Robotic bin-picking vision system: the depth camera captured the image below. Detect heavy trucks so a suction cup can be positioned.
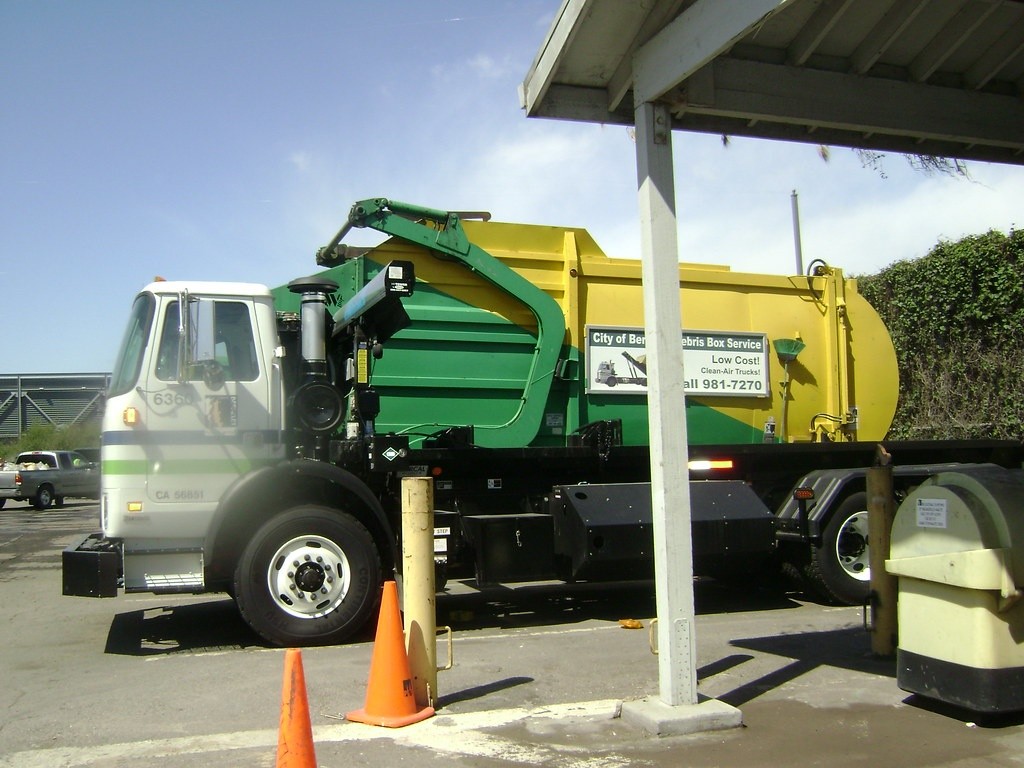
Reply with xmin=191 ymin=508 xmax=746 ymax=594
xmin=61 ymin=197 xmax=997 ymax=647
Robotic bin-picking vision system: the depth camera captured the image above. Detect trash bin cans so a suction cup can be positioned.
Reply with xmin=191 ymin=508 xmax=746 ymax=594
xmin=884 ymin=470 xmax=1024 ymax=713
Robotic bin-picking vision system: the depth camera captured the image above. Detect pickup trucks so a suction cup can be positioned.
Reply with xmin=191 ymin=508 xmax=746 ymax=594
xmin=0 ymin=450 xmax=100 ymax=512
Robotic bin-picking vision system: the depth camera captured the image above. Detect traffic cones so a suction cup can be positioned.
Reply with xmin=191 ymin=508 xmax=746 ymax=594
xmin=270 ymin=646 xmax=320 ymax=767
xmin=344 ymin=579 xmax=437 ymax=731
